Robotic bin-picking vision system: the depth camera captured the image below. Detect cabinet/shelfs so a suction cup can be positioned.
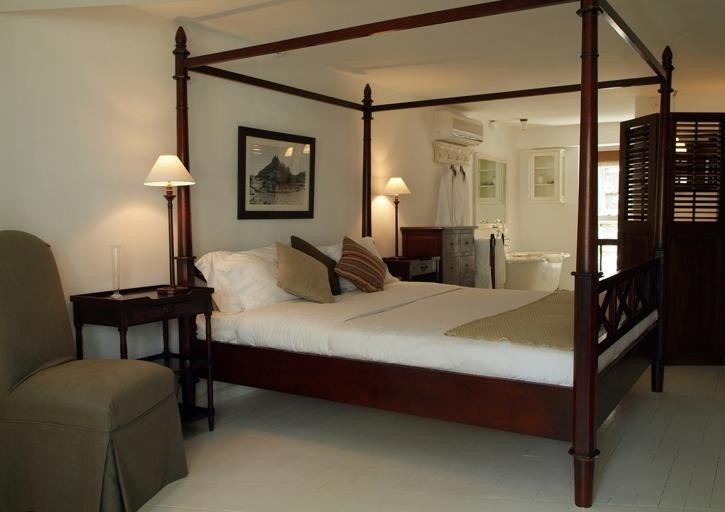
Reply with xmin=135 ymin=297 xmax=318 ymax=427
xmin=400 ymin=225 xmax=478 ymax=287
xmin=528 ymin=148 xmax=566 ymax=203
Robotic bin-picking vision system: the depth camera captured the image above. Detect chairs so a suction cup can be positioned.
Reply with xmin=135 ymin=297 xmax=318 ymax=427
xmin=0 ymin=231 xmax=188 ymax=512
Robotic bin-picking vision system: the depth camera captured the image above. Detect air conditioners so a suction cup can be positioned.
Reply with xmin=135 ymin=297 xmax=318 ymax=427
xmin=433 ymin=110 xmax=483 ymax=146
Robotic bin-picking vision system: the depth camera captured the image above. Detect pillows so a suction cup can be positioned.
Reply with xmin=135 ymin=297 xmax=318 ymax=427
xmin=291 ymin=234 xmax=341 ymax=295
xmin=276 ymin=241 xmax=334 ymax=304
xmin=194 ymin=242 xmax=299 ymax=313
xmin=319 ymin=236 xmax=399 ymax=291
xmin=334 ymin=236 xmax=387 ymax=292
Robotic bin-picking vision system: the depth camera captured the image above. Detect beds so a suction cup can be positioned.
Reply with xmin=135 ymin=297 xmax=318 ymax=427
xmin=172 ymin=0 xmax=674 ymax=507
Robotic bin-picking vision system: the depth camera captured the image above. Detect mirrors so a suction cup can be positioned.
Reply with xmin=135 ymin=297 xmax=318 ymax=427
xmin=473 ymin=152 xmax=509 ymax=226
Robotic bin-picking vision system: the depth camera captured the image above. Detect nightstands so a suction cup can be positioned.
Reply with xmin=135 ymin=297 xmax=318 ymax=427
xmin=382 ymin=256 xmax=440 ymax=283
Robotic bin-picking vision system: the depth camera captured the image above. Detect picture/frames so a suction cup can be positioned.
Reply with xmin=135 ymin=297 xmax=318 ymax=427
xmin=238 ymin=125 xmax=315 ymax=219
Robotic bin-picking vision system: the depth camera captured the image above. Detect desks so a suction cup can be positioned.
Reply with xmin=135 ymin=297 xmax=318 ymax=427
xmin=69 ymin=284 xmax=215 ymax=431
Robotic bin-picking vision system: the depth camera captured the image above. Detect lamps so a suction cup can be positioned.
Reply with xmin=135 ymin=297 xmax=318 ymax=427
xmin=384 ymin=177 xmax=411 ymax=259
xmin=145 ymin=155 xmax=195 ymax=293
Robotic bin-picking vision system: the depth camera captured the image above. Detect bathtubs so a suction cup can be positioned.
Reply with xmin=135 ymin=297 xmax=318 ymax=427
xmin=504 ymin=251 xmax=571 ymax=290
